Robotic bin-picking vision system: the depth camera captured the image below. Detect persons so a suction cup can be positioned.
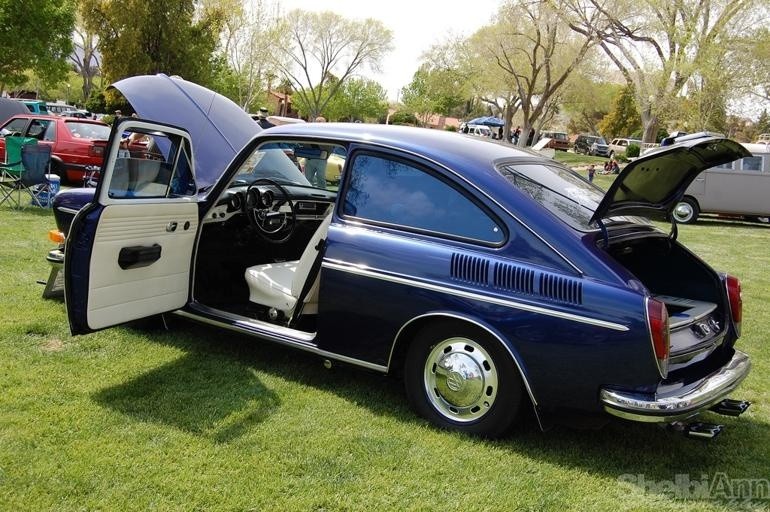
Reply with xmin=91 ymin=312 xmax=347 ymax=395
xmin=120 ymin=130 xmax=166 ymax=161
xmin=497 ymin=126 xmax=536 ymax=146
xmin=587 ymin=161 xmax=620 ymax=183
xmin=112 ymin=110 xmax=122 ymax=126
xmin=304 ymin=116 xmax=331 ymax=189
xmin=255 ymin=107 xmax=276 ymax=129
xmin=464 ymin=125 xmax=469 ymax=134
xmin=459 ymin=128 xmax=463 ymax=133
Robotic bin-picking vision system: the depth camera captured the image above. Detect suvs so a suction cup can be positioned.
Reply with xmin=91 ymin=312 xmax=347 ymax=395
xmin=607 ymin=138 xmax=642 ymax=159
xmin=634 ymin=138 xmax=769 ymax=225
xmin=573 ymin=134 xmax=609 ymax=157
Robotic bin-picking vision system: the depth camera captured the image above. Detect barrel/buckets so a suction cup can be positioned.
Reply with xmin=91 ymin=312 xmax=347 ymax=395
xmin=33 ymin=190 xmax=49 ymax=206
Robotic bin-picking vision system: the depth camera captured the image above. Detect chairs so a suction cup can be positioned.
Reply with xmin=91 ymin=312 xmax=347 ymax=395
xmin=244 ymin=210 xmax=334 ymax=324
xmin=0 ymin=136 xmax=50 ymax=213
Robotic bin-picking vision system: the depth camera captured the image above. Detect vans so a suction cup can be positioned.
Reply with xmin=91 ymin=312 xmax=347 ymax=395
xmin=541 ymin=131 xmax=571 ymax=151
xmin=462 ymin=125 xmax=493 ymax=139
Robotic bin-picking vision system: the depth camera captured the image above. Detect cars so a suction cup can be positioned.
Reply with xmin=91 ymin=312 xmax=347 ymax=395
xmin=49 ymin=73 xmax=756 ymax=440
xmin=20 ymin=99 xmax=88 ymax=119
xmin=2 ymin=113 xmax=130 ymax=187
xmin=2 ymin=98 xmax=46 ymax=166
xmin=126 ymin=130 xmax=165 ymax=162
